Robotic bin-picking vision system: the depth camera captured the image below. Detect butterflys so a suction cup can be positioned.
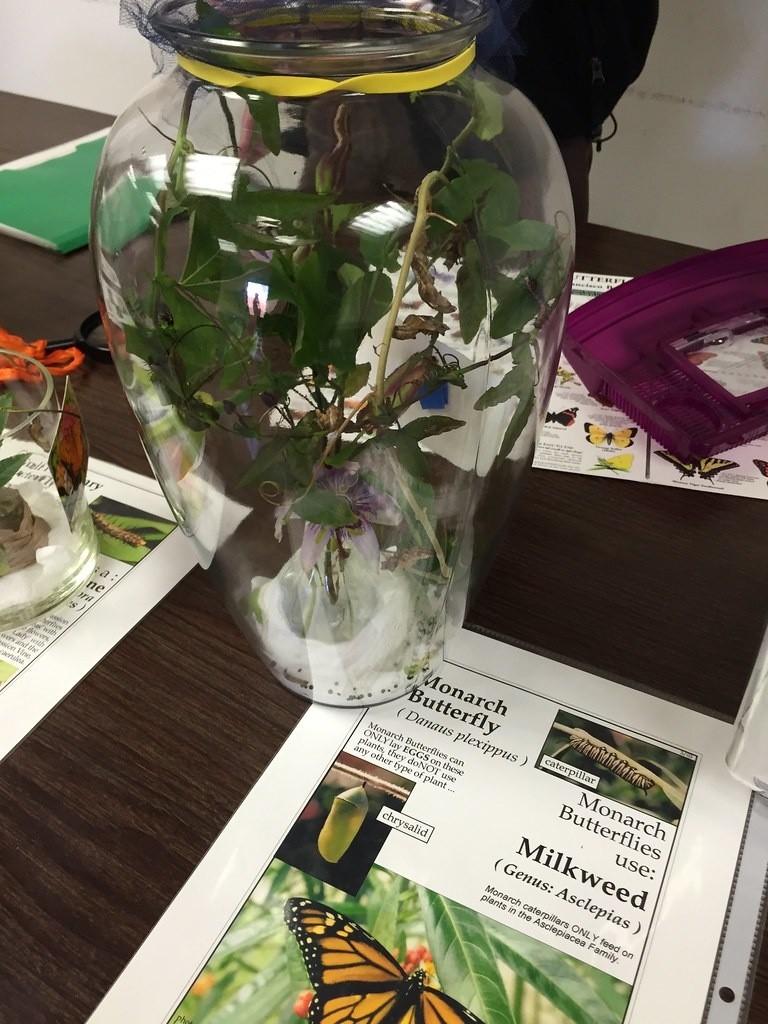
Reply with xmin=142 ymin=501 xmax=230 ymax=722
xmin=283 ymin=896 xmax=486 ymax=1024
xmin=541 ymin=335 xmax=767 ymax=485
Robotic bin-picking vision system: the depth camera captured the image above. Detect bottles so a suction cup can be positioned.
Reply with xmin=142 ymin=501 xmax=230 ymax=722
xmin=89 ymin=5 xmax=576 ymax=708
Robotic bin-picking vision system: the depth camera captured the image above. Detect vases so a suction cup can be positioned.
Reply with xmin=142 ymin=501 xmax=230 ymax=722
xmin=83 ymin=0 xmax=582 ymax=714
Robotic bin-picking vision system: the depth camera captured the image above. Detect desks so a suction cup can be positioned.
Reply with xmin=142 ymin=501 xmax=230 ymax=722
xmin=0 ymin=86 xmax=768 ymax=1024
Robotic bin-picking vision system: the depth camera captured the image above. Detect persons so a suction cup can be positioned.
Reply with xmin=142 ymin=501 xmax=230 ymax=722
xmin=299 ymin=0 xmax=660 ymax=223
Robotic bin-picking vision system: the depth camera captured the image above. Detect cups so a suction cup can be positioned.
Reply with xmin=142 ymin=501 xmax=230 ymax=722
xmin=0 ymin=350 xmax=99 ymax=632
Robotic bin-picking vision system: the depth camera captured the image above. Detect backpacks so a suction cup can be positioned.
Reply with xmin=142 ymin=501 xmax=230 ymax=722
xmin=427 ymin=0 xmax=660 ymax=153
